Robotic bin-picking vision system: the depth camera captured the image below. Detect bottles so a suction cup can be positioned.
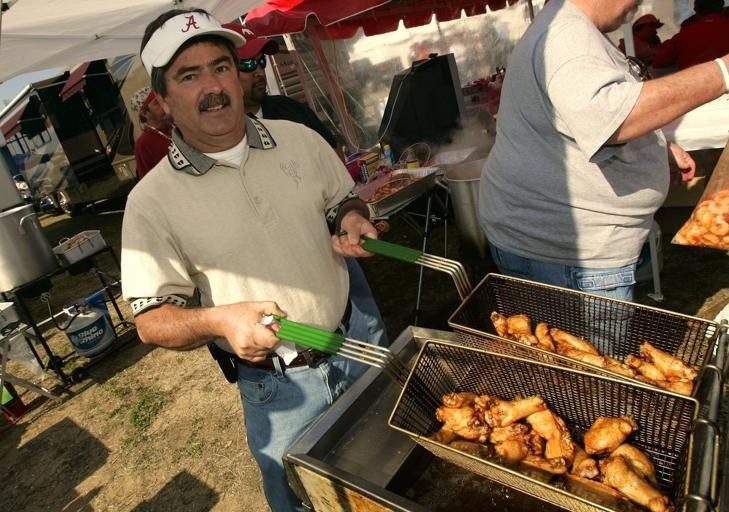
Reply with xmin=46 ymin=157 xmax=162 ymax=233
xmin=407 ymin=159 xmax=419 ymax=169
xmin=384 ymin=145 xmax=396 ymax=167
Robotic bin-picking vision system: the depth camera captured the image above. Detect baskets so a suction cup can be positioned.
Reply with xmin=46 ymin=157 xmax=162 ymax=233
xmin=388 ymin=340 xmax=700 ymax=512
xmin=447 ymin=271 xmax=720 ymax=399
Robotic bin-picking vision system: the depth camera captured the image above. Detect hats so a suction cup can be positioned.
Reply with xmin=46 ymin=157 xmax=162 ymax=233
xmin=222 ymin=23 xmax=279 ymax=60
xmin=140 ymin=12 xmax=247 ymax=79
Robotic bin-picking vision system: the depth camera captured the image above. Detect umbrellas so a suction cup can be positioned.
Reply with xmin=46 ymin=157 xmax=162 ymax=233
xmin=245 ymin=0 xmax=519 ymax=152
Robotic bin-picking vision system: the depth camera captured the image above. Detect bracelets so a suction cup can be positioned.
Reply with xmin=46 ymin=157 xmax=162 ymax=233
xmin=715 ymin=57 xmax=729 ymax=93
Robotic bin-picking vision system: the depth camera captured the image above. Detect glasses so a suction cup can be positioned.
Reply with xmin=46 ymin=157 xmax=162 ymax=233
xmin=237 ymin=56 xmax=266 ymax=72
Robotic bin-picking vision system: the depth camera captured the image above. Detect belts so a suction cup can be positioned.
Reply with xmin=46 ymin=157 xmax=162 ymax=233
xmin=236 ymin=294 xmax=352 ymax=369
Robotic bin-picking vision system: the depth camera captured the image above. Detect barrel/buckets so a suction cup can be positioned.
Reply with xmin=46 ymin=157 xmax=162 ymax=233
xmin=66 ymin=310 xmax=114 ymax=359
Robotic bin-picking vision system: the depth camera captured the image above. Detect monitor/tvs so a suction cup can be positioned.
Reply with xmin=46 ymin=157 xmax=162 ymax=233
xmin=377 ymin=53 xmax=476 ymax=160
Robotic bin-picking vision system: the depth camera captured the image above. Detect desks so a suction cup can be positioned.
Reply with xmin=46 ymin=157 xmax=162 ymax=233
xmin=4 ymin=244 xmax=170 ymax=391
xmin=354 ymin=172 xmax=477 ymax=325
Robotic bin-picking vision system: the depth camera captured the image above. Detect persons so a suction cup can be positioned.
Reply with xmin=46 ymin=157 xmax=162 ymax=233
xmin=479 ymin=0 xmax=729 ymax=356
xmin=130 ymin=86 xmax=177 ymax=180
xmin=618 ymin=14 xmax=664 ymax=63
xmin=120 ymin=8 xmax=377 ymax=512
xmin=221 ymin=23 xmax=345 ymax=166
xmin=652 ymin=0 xmax=729 ymax=70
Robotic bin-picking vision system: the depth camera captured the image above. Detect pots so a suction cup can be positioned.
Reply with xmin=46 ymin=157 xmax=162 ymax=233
xmin=0 ymin=151 xmax=60 ymax=293
xmin=434 ymin=158 xmax=498 ymax=267
